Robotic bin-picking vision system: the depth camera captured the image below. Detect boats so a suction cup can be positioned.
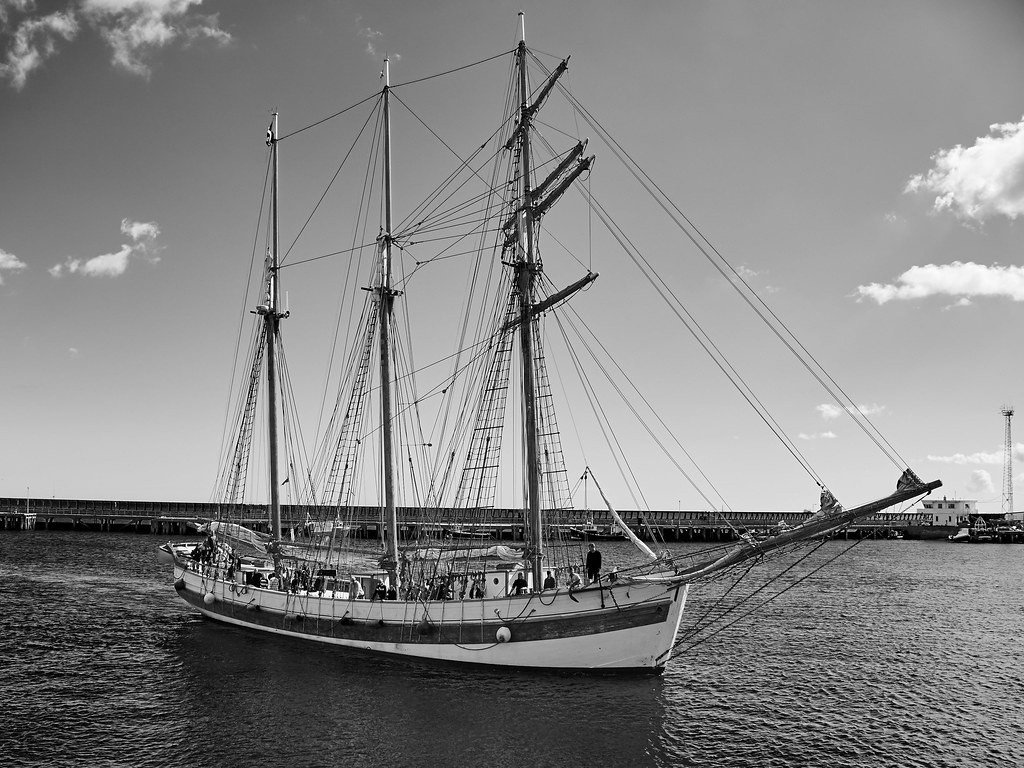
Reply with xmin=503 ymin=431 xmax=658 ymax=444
xmin=569 ymin=509 xmax=626 ymax=542
xmin=447 ymin=528 xmax=491 ymax=540
xmin=305 ymin=507 xmax=350 ymax=538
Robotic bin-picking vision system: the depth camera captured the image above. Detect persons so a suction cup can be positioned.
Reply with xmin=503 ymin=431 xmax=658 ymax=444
xmin=251 ymin=563 xmax=326 ymax=594
xmin=566 ymin=567 xmax=582 ymax=587
xmin=507 ymin=572 xmax=528 ymax=597
xmin=349 ymin=577 xmax=365 ymax=600
xmin=544 ymin=571 xmax=555 ymax=589
xmin=586 ymin=544 xmax=602 ymax=584
xmin=189 ymin=529 xmax=241 ymax=581
xmin=370 ymin=578 xmax=482 ymax=602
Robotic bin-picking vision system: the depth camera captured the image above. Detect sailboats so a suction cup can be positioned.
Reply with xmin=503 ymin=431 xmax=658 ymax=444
xmin=154 ymin=8 xmax=946 ymax=679
